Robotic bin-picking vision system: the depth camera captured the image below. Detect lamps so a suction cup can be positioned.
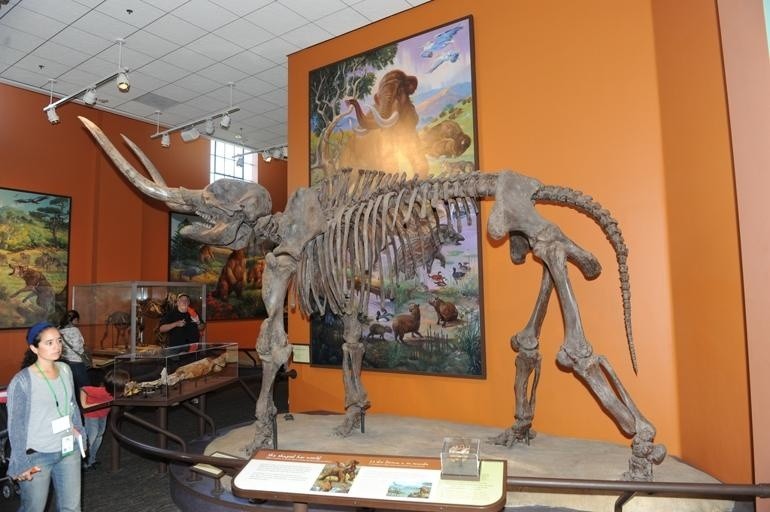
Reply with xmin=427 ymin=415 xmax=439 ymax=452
xmin=38 ymin=32 xmax=134 ymax=126
xmin=147 ymin=76 xmax=245 ymax=151
xmin=230 ymin=129 xmax=288 ymax=170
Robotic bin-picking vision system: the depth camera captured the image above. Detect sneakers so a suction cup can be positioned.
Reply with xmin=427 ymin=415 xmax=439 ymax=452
xmin=82 ymin=460 xmax=100 ymax=472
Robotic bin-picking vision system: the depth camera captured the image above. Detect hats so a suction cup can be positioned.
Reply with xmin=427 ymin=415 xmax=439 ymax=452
xmin=176 ymin=292 xmax=189 ymax=300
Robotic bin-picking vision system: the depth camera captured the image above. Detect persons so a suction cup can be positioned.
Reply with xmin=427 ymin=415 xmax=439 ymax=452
xmin=78 ymin=369 xmax=131 ymax=472
xmin=56 ymin=309 xmax=89 ymax=392
xmin=158 ymin=294 xmax=206 ymax=406
xmin=8 ymin=321 xmax=89 ymax=512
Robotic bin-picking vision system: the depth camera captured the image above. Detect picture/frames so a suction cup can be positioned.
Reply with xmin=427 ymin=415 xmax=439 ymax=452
xmin=308 ymin=14 xmax=487 ymax=380
xmin=166 ymin=209 xmax=290 ymax=324
xmin=0 ymin=186 xmax=73 ymax=330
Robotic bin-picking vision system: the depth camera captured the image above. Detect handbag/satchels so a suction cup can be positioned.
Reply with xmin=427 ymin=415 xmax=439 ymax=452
xmin=80 ymin=351 xmax=93 ymax=369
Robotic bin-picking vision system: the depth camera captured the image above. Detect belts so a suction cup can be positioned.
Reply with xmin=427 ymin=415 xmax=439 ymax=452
xmin=26 ymin=448 xmax=37 ymax=456
